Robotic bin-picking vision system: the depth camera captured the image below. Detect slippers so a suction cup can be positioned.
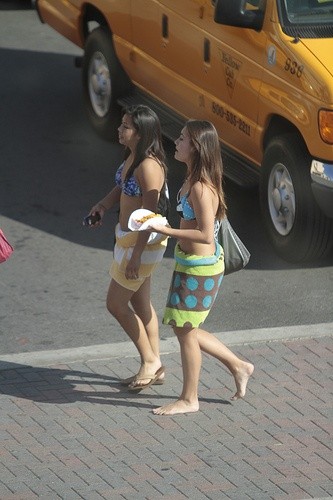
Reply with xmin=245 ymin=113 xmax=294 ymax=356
xmin=126 ymin=364 xmax=166 ymax=391
xmin=119 ymin=372 xmax=165 ymax=386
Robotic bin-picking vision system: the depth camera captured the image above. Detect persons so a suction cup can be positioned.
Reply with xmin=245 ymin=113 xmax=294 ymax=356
xmin=87 ymin=105 xmax=169 ymax=392
xmin=146 ymin=119 xmax=254 ymax=416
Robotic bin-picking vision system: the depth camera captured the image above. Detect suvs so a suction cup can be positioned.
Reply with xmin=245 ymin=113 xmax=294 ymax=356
xmin=37 ymin=0 xmax=333 ymax=264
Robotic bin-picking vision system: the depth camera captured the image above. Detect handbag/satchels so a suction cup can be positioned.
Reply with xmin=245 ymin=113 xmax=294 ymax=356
xmin=217 ymin=213 xmax=252 ymax=275
xmin=0 ymin=227 xmax=13 ymax=264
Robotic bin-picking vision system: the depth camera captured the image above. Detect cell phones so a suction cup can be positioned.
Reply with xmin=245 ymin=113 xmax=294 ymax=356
xmin=84 ymin=212 xmax=101 ymax=225
